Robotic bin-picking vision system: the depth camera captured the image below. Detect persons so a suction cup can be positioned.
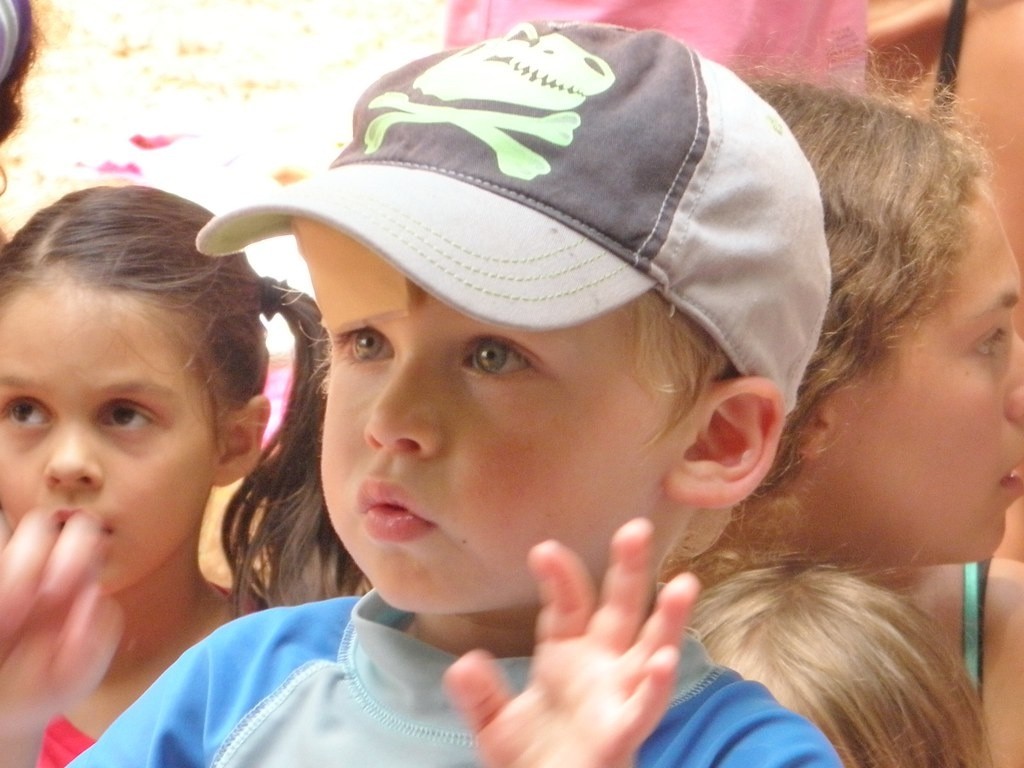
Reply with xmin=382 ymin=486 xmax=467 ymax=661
xmin=0 ymin=186 xmax=371 ymax=768
xmin=680 ymin=558 xmax=987 ymax=768
xmin=660 ymin=71 xmax=1024 ymax=768
xmin=63 ymin=21 xmax=845 ymax=768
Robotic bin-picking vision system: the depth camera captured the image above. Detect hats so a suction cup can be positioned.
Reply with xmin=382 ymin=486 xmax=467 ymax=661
xmin=191 ymin=19 xmax=833 ymax=420
